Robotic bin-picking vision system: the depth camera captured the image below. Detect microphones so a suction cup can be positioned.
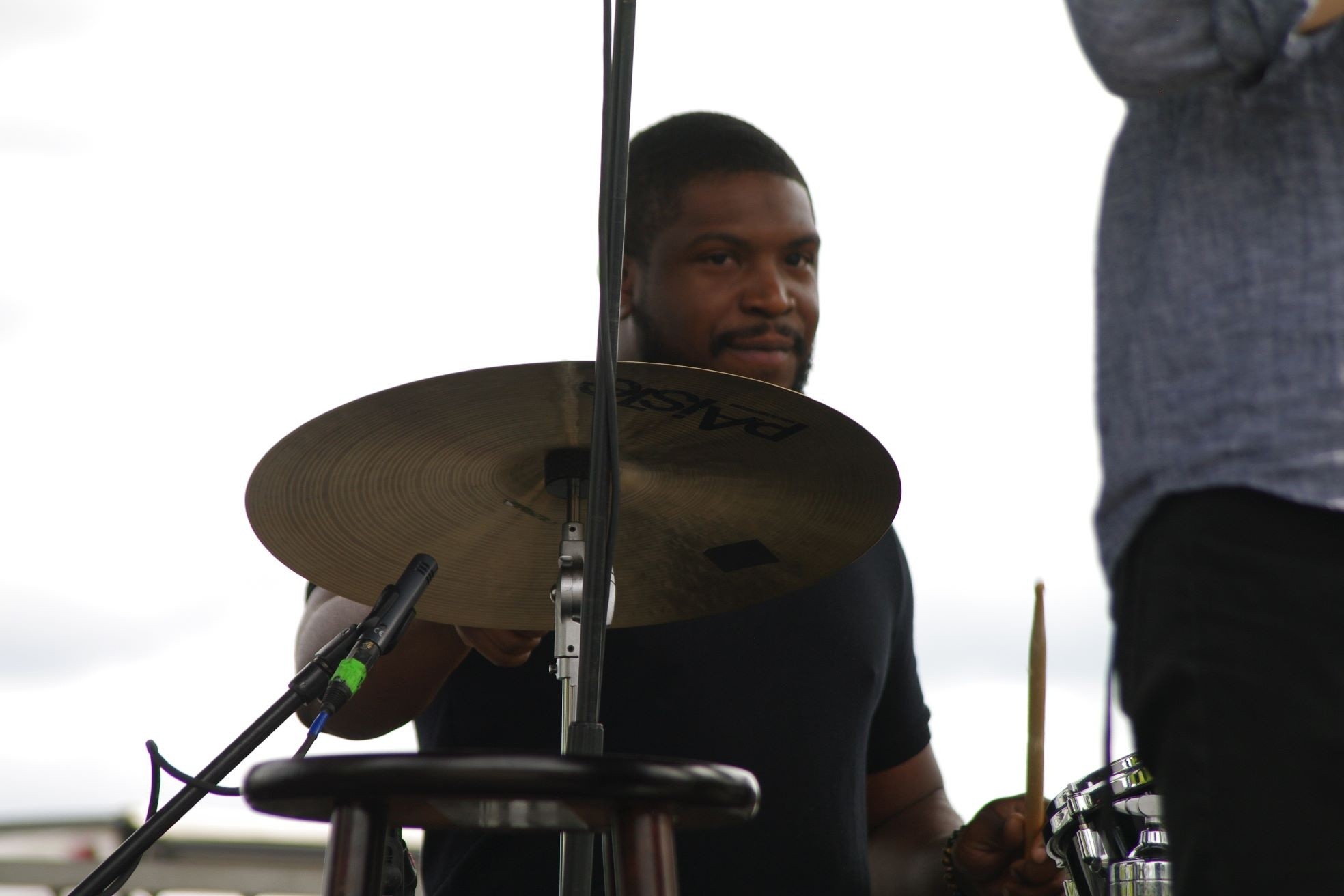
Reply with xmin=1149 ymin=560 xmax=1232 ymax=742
xmin=319 ymin=552 xmax=438 ymax=718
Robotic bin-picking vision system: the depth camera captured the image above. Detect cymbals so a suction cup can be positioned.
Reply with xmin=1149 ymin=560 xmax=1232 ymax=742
xmin=242 ymin=358 xmax=904 ymax=634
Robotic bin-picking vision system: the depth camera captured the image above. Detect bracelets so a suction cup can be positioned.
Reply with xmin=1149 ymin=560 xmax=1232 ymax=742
xmin=941 ymin=825 xmax=970 ymax=896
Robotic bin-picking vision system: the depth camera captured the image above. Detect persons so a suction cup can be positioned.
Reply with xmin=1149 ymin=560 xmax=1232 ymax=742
xmin=295 ymin=111 xmax=1070 ymax=896
xmin=1065 ymin=0 xmax=1344 ymax=896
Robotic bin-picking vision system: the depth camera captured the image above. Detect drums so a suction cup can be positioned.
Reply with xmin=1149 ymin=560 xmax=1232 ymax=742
xmin=1042 ymin=749 xmax=1178 ymax=896
